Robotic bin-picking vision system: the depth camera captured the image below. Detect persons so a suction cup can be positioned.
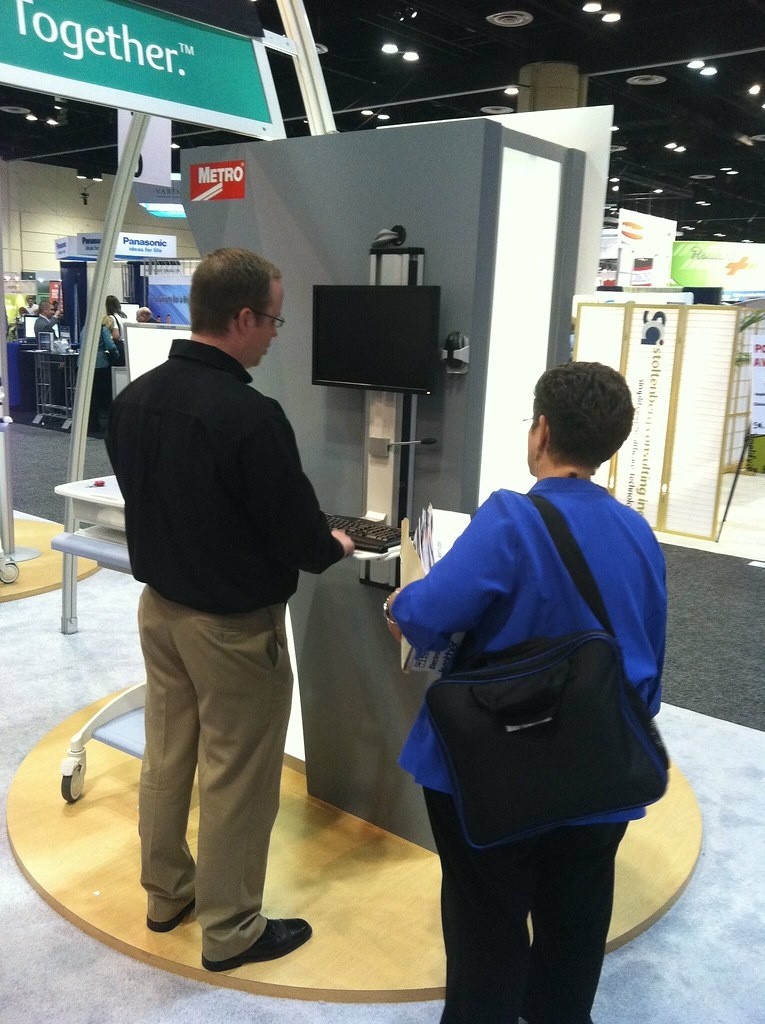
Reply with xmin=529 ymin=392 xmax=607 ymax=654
xmin=18 ymin=307 xmax=31 ymax=322
xmin=25 ymin=298 xmax=39 ymax=315
xmin=136 ymin=307 xmax=159 ymax=323
xmin=157 ymin=316 xmax=162 ymax=322
xmin=387 ymin=362 xmax=668 ymax=1024
xmin=76 ymin=295 xmax=130 ymax=431
xmin=105 ymin=246 xmax=355 ymax=972
xmin=34 ymin=301 xmax=64 ymax=420
xmin=166 ymin=315 xmax=171 ymax=323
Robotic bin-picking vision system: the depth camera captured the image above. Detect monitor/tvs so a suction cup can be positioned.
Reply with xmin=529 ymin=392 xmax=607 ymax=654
xmin=23 ymin=315 xmax=61 ymax=341
xmin=310 ymin=284 xmax=440 ymax=396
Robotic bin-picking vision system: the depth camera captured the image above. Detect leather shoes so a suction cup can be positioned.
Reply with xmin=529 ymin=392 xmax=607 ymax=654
xmin=201 ymin=918 xmax=313 ymax=972
xmin=147 ymin=898 xmax=197 ymax=932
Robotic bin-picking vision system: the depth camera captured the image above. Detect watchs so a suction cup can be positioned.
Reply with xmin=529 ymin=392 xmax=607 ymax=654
xmin=383 ymin=593 xmax=398 ymax=625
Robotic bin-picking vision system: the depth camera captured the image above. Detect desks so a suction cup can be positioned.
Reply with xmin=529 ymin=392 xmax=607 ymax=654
xmin=22 ymin=348 xmax=79 ymax=430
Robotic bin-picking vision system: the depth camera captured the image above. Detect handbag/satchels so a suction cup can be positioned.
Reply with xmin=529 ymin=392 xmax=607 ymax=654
xmin=109 ymin=341 xmax=125 ymax=366
xmin=425 ymin=492 xmax=671 ymax=848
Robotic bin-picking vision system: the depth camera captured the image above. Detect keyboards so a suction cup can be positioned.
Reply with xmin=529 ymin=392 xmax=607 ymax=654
xmin=323 ymin=512 xmax=415 ymax=554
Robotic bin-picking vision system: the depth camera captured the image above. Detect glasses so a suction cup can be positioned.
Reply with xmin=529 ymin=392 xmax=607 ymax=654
xmin=523 ymin=417 xmax=540 ymax=431
xmin=234 ymin=307 xmax=285 ymax=327
